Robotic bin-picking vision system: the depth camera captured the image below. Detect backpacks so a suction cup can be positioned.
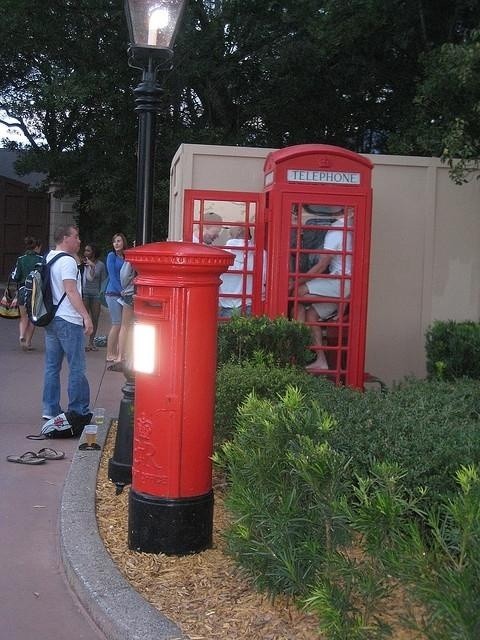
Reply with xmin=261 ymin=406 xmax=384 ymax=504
xmin=24 ymin=251 xmax=78 ymax=327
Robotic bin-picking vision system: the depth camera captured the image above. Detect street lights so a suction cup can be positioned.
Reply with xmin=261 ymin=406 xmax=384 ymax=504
xmin=105 ymin=1 xmax=192 ymax=488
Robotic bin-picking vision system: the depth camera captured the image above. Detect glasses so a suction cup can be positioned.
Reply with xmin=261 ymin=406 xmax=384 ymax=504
xmin=79 ymin=443 xmax=101 ymax=450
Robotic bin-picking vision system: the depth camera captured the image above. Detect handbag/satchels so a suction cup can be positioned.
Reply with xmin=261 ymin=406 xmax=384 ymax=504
xmin=98 ymin=291 xmax=108 ymax=307
xmin=40 ymin=412 xmax=92 ymax=439
xmin=0 ymin=289 xmax=20 ymax=318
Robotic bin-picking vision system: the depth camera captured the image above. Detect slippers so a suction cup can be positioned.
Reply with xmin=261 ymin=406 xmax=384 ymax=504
xmin=7 ymin=452 xmax=45 ymax=465
xmin=36 ymin=447 xmax=65 ymax=460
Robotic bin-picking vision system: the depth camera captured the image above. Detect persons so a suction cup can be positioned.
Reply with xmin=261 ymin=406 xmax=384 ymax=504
xmin=7 ymin=233 xmax=135 ymax=363
xmin=179 ymin=203 xmax=353 ymax=371
xmin=39 ymin=225 xmax=94 ymax=423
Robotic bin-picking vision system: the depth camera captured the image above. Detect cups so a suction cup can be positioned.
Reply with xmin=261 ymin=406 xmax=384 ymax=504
xmin=84 ymin=424 xmax=98 ymax=447
xmin=93 ymin=408 xmax=105 ymax=425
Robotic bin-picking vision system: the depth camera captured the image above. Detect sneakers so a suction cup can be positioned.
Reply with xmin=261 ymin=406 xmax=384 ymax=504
xmin=42 ymin=415 xmax=54 ymax=420
xmin=20 ymin=337 xmax=27 ymax=351
